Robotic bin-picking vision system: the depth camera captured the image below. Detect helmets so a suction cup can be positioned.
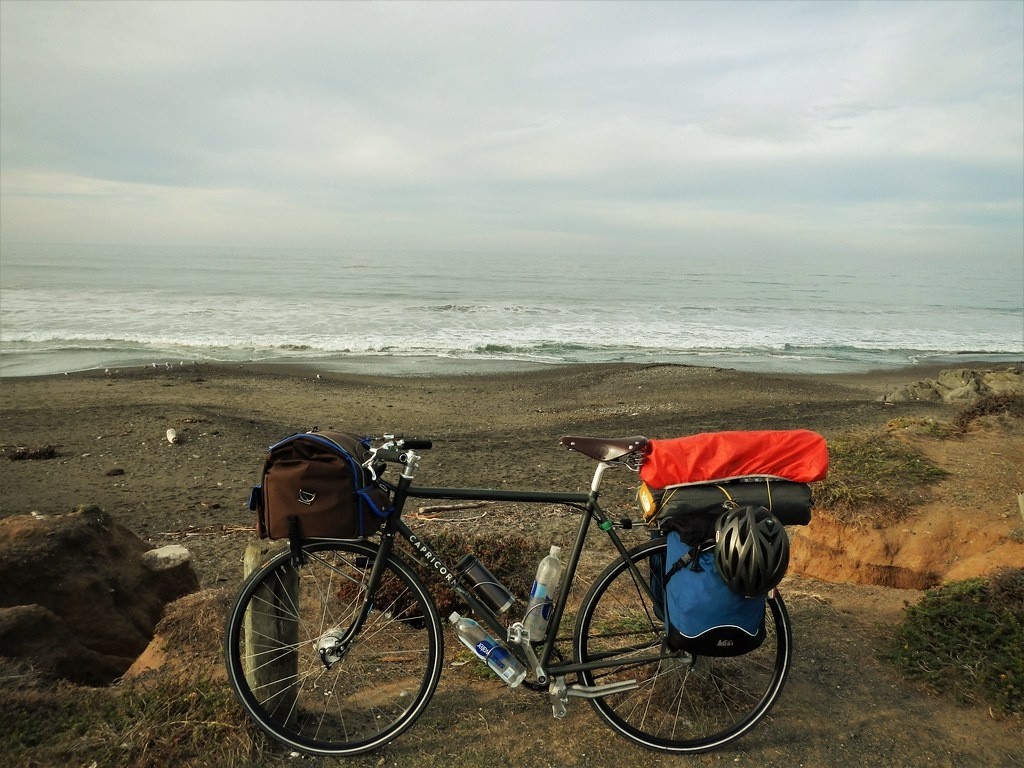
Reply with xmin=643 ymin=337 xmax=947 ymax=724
xmin=714 ymin=505 xmax=789 ymax=598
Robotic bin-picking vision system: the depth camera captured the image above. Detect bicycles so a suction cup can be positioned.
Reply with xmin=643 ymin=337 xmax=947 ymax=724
xmin=220 ymin=435 xmax=795 ymax=757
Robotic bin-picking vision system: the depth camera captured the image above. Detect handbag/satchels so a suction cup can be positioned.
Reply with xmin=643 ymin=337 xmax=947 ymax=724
xmin=641 ymin=429 xmax=829 ymax=526
xmin=249 ymin=426 xmax=394 ymax=540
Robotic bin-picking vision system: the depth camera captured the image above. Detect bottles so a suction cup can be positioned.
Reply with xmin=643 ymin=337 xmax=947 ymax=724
xmin=524 ymin=546 xmax=562 ymax=642
xmin=449 ymin=612 xmax=527 ymax=688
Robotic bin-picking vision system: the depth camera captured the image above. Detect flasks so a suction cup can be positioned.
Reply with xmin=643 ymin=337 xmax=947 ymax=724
xmin=454 ymin=553 xmax=515 ymax=617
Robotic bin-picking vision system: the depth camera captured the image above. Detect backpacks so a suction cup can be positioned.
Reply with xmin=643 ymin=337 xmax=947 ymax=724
xmin=650 ymin=524 xmax=766 ymax=656
